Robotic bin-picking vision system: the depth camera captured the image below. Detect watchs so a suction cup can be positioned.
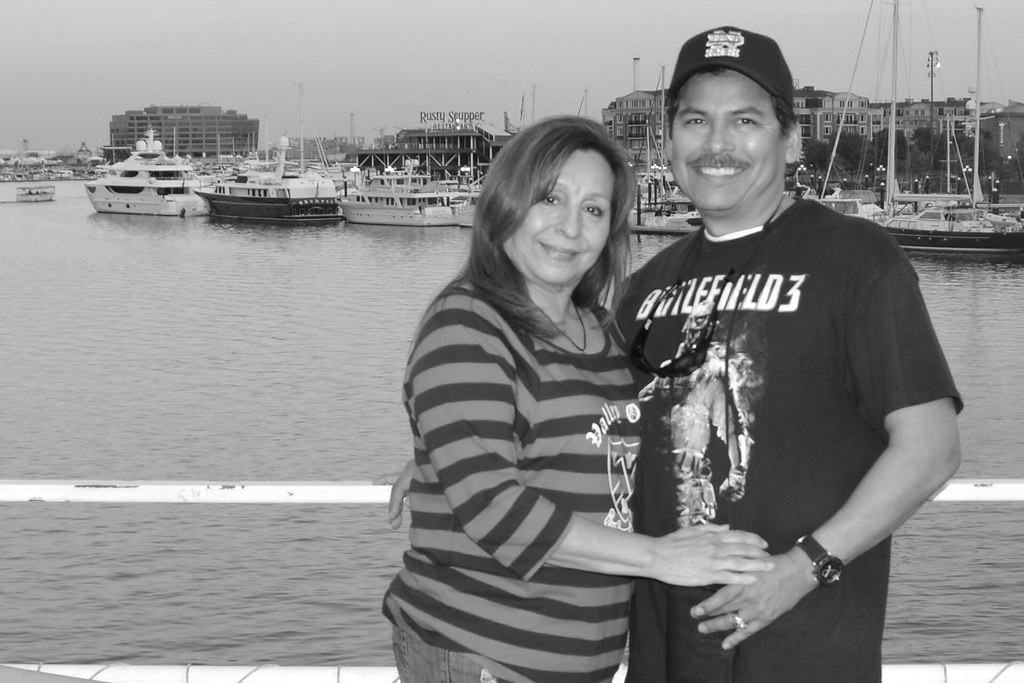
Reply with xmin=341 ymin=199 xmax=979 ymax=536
xmin=796 ymin=535 xmax=844 ymax=587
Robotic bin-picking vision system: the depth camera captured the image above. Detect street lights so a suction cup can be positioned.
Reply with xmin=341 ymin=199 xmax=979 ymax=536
xmin=925 ymin=49 xmax=941 ymax=167
xmin=633 ymin=57 xmax=641 ymax=92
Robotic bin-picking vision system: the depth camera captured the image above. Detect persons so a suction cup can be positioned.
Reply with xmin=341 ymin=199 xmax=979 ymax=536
xmin=380 ymin=112 xmax=773 ymax=683
xmin=388 ymin=24 xmax=963 ymax=682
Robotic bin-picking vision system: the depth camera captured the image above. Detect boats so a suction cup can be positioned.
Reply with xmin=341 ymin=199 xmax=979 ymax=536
xmin=334 ymin=159 xmax=469 ymax=226
xmin=84 ymin=124 xmax=212 ymax=217
xmin=191 ymin=80 xmax=343 ymax=223
xmin=0 ymin=140 xmax=111 ymax=183
xmin=14 ymin=184 xmax=56 ymax=204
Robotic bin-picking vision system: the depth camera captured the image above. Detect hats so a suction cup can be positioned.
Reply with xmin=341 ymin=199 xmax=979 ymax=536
xmin=667 ymin=26 xmax=794 ymax=109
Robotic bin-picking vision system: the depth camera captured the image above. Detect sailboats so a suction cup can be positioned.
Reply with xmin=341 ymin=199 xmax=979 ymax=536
xmin=444 ymin=0 xmax=1024 ymax=257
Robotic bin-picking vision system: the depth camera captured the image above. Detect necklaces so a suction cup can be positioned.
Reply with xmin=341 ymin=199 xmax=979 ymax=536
xmin=528 ymin=298 xmax=587 ymax=355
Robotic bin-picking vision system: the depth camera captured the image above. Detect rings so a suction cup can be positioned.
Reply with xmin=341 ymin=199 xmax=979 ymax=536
xmin=730 ymin=613 xmax=745 ymax=630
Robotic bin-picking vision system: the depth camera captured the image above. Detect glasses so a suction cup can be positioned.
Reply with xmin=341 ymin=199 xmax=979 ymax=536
xmin=629 ymin=266 xmax=735 ymax=379
